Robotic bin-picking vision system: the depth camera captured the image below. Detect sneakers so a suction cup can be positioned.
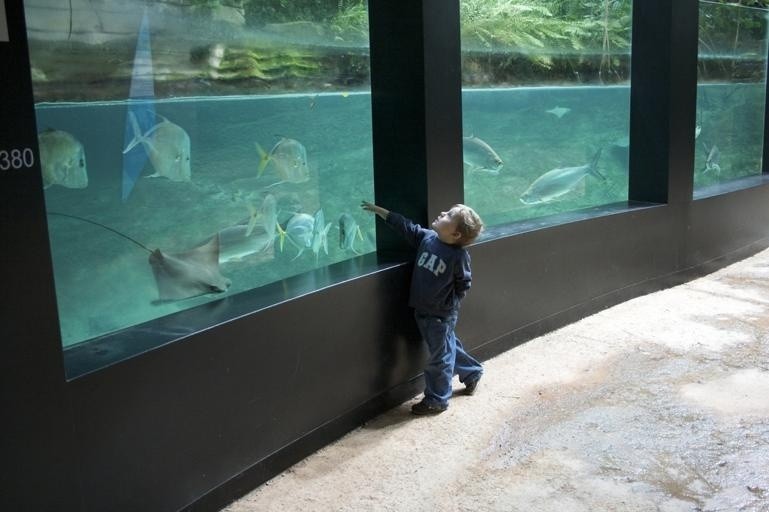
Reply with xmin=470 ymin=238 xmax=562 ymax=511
xmin=465 ymin=378 xmax=481 ymax=395
xmin=412 ymin=401 xmax=446 ymax=414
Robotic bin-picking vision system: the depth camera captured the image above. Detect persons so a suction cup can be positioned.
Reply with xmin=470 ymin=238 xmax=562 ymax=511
xmin=359 ymin=196 xmax=484 ymax=415
